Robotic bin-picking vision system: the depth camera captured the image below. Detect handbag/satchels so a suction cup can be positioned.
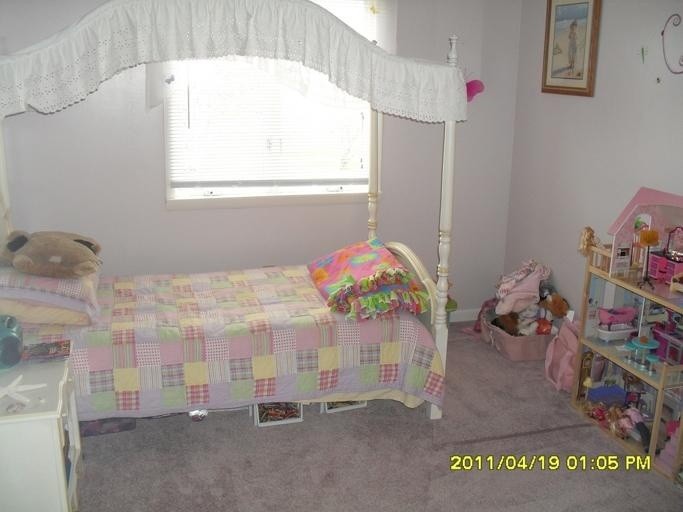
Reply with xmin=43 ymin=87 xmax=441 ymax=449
xmin=545 ymin=318 xmax=579 ymax=394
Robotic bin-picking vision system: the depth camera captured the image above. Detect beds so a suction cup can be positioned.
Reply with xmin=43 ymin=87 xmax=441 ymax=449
xmin=0 ymin=241 xmax=445 ymax=418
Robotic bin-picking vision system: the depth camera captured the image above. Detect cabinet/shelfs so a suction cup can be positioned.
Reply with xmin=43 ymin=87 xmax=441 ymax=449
xmin=571 ymin=185 xmax=683 ymax=481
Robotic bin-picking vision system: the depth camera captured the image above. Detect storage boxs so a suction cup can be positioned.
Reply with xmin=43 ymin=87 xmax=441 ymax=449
xmin=481 ymin=313 xmax=557 ymax=362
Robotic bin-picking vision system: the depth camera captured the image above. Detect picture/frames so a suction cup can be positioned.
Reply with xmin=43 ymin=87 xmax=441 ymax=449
xmin=541 ymin=0 xmax=601 ymax=97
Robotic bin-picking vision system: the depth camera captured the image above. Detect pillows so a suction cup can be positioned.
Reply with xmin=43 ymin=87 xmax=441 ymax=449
xmin=0 ymin=231 xmax=104 ymax=326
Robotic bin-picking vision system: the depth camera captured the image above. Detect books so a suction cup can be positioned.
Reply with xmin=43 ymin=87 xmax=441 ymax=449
xmin=21 ymin=339 xmax=74 ymax=360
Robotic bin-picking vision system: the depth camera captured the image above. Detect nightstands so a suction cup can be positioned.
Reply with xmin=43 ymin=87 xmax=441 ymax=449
xmin=0 ymin=341 xmax=82 ymax=512
